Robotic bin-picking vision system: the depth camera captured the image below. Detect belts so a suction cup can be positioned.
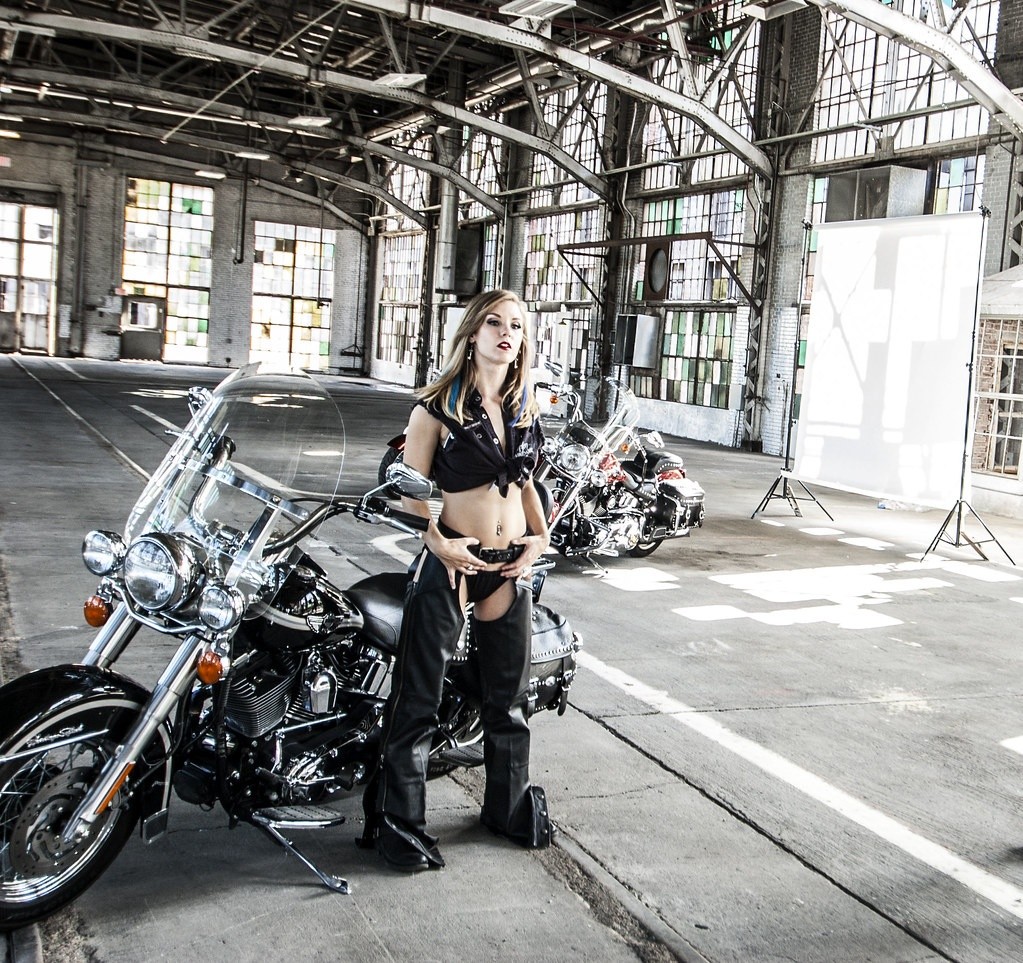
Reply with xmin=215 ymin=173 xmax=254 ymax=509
xmin=438 ymin=515 xmax=534 ymax=564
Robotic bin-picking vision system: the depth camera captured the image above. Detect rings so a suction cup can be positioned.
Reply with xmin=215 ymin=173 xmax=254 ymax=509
xmin=468 ymin=564 xmax=474 ymax=571
xmin=522 ymin=569 xmax=525 ymax=573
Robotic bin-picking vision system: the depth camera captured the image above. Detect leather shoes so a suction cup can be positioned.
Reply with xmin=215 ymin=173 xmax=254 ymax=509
xmin=484 ymin=822 xmax=557 ymax=840
xmin=379 ymin=829 xmax=430 ymax=872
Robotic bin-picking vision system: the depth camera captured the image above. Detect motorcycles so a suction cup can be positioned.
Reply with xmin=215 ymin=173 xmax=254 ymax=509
xmin=0 ymin=359 xmax=584 ymax=934
xmin=378 ymin=361 xmax=706 ymax=574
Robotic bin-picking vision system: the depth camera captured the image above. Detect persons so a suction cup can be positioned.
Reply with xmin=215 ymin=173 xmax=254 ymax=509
xmin=354 ymin=290 xmax=553 ymax=871
